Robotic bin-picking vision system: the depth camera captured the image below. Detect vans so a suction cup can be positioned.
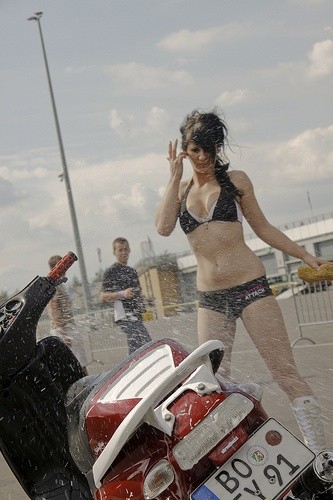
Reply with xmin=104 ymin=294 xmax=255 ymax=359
xmin=266 ymin=270 xmax=304 ymax=295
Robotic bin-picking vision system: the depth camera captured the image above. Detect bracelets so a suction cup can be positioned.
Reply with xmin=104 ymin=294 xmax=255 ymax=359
xmin=116 ymin=290 xmax=123 ymax=301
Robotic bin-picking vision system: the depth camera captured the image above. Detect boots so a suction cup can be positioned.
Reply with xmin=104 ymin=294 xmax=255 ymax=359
xmin=289 ymin=395 xmax=326 ymax=456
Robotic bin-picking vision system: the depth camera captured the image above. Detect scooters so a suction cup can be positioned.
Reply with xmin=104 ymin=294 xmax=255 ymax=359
xmin=0 ymin=251 xmax=333 ymax=499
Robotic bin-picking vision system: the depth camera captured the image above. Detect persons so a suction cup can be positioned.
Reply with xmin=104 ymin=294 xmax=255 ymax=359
xmin=153 ymin=109 xmax=333 ymax=456
xmin=100 ymin=237 xmax=153 ymax=354
xmin=47 ymin=255 xmax=75 ymax=352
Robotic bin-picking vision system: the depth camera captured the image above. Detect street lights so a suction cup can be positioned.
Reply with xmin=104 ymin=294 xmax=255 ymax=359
xmin=26 ymin=9 xmax=93 ymax=313
